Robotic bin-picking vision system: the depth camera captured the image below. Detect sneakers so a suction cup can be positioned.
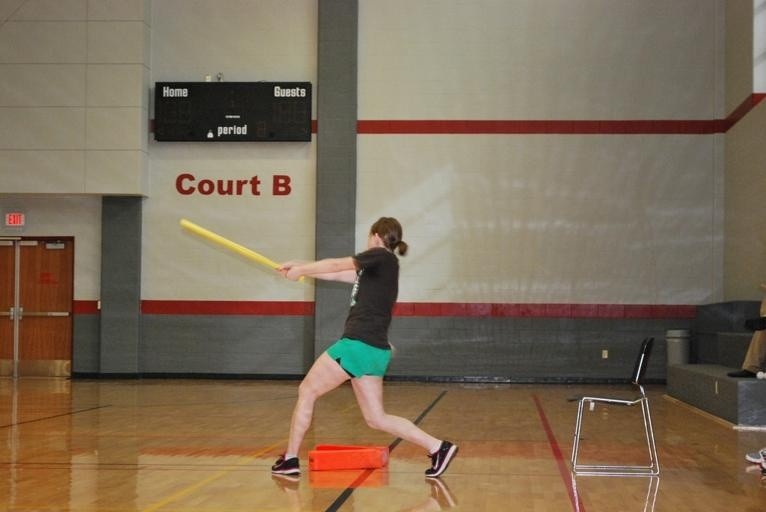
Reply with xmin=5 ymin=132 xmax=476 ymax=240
xmin=425 ymin=441 xmax=459 ymax=477
xmin=744 ymin=317 xmax=766 ymax=330
xmin=745 ymin=449 xmax=766 ymax=469
xmin=272 ymin=455 xmax=301 ymax=475
xmin=727 ymin=369 xmax=756 ymax=378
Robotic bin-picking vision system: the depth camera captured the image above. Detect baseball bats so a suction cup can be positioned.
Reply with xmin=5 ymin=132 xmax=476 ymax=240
xmin=179 ymin=217 xmax=305 ymax=282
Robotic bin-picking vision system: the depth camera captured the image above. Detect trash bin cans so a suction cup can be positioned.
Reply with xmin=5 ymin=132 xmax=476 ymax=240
xmin=666 ymin=330 xmax=691 ymax=366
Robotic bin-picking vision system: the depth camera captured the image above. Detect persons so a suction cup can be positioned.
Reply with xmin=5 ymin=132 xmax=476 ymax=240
xmin=270 ymin=474 xmax=459 ymax=511
xmin=271 ymin=218 xmax=459 ymax=478
xmin=727 ymin=283 xmax=766 ymax=378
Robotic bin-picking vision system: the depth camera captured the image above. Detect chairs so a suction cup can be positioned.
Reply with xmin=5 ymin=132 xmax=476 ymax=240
xmin=567 ymin=336 xmax=659 ymax=476
